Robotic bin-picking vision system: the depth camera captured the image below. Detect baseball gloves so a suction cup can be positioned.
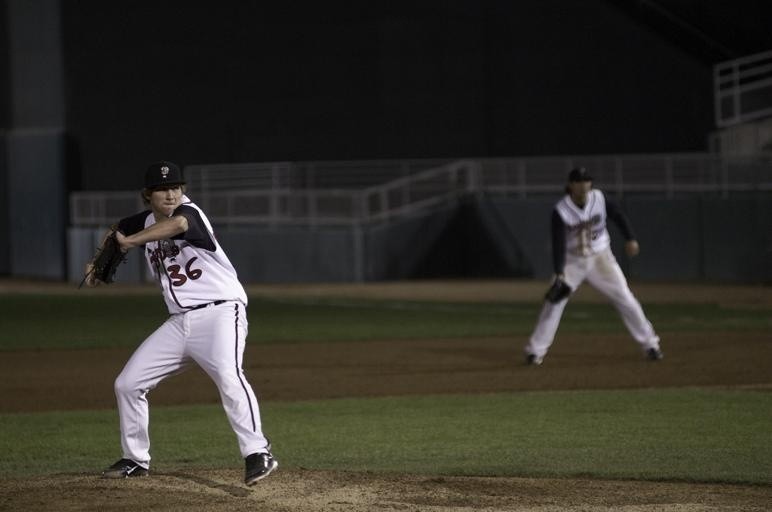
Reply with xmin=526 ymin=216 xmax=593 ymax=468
xmin=79 ymin=231 xmax=128 ymax=289
xmin=546 ymin=278 xmax=572 ymax=304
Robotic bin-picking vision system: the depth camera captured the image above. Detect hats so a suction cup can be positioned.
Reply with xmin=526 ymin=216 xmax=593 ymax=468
xmin=145 ymin=162 xmax=186 ymax=187
xmin=570 ymin=167 xmax=592 ymax=180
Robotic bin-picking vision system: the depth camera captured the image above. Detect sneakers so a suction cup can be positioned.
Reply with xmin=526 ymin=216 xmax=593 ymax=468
xmin=647 ymin=348 xmax=663 ymax=360
xmin=103 ymin=458 xmax=148 ymax=479
xmin=527 ymin=354 xmax=543 ymax=367
xmin=245 ymin=453 xmax=278 ymax=485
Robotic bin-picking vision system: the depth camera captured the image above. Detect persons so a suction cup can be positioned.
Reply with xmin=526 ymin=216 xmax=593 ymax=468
xmin=82 ymin=160 xmax=279 ymax=487
xmin=517 ymin=164 xmax=664 ymax=365
